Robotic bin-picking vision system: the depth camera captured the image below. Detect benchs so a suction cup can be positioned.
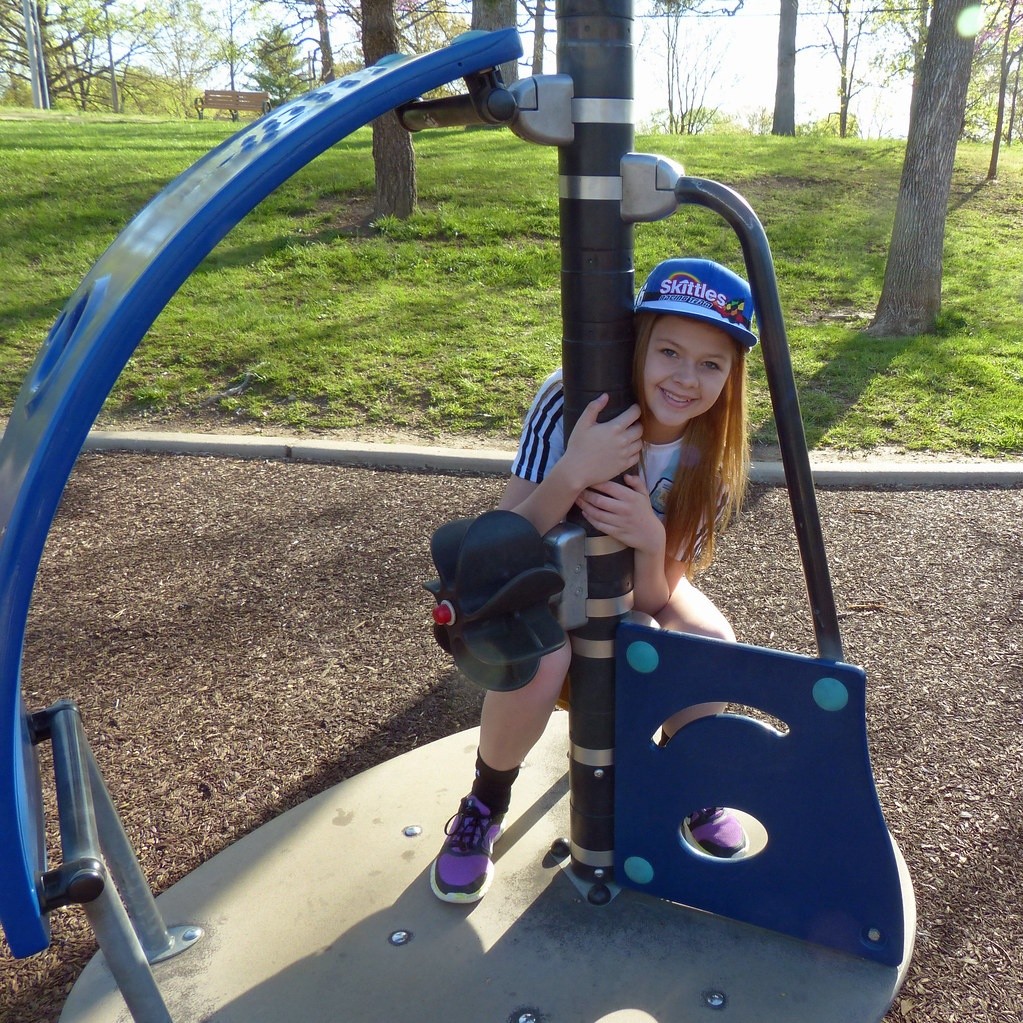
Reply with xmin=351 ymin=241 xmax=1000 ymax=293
xmin=194 ymin=90 xmax=271 ymax=122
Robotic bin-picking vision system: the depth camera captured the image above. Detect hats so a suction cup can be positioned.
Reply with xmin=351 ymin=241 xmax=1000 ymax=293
xmin=633 ymin=257 xmax=758 ymax=354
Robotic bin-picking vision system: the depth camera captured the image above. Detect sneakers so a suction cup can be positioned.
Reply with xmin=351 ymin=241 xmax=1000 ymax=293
xmin=430 ymin=790 xmax=505 ymax=903
xmin=681 ymin=807 xmax=750 ymax=861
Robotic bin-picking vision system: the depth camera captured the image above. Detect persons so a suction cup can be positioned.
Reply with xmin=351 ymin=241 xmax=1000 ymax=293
xmin=431 ymin=256 xmax=758 ymax=904
